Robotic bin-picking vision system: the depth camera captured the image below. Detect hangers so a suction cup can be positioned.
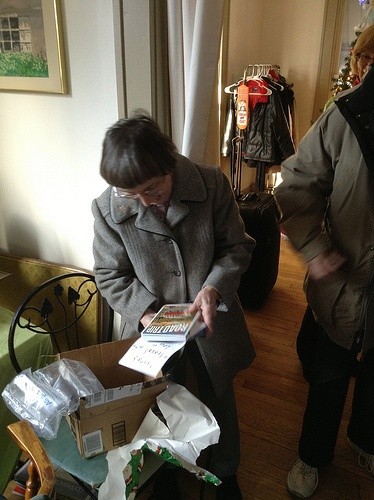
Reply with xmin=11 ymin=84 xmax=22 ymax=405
xmin=224 ymin=62 xmax=296 ymax=96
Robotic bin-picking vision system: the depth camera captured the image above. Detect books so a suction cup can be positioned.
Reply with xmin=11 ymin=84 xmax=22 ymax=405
xmin=141 ymin=303 xmax=202 ymax=342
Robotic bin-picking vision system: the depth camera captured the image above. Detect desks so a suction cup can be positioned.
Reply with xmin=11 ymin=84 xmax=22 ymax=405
xmin=1 ymin=305 xmax=54 ymax=494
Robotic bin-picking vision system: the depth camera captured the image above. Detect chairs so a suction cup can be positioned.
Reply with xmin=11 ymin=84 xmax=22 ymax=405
xmin=0 ymin=272 xmax=214 ymax=500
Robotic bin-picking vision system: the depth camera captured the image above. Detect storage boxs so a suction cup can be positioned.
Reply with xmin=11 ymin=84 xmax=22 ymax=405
xmin=56 ymin=334 xmax=168 ymax=460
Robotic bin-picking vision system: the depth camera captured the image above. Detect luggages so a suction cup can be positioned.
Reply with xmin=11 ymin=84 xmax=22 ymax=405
xmin=231 ymin=135 xmax=280 ymax=307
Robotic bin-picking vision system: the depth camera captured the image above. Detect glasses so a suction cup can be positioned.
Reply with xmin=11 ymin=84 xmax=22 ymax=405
xmin=121 ymin=175 xmax=166 ymax=200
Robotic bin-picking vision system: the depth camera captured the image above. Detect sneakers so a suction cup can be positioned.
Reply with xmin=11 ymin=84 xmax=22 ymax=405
xmin=288 ymin=456 xmax=319 ymax=499
xmin=346 ymin=438 xmax=373 ymax=475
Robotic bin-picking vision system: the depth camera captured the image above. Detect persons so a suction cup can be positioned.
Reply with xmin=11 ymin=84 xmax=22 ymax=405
xmin=322 ymin=23 xmax=374 ymax=111
xmin=274 ymin=62 xmax=374 ymax=498
xmin=93 ymin=114 xmax=257 ymax=500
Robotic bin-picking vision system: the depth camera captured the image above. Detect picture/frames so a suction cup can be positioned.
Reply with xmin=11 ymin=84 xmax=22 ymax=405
xmin=0 ymin=0 xmax=69 ymax=96
